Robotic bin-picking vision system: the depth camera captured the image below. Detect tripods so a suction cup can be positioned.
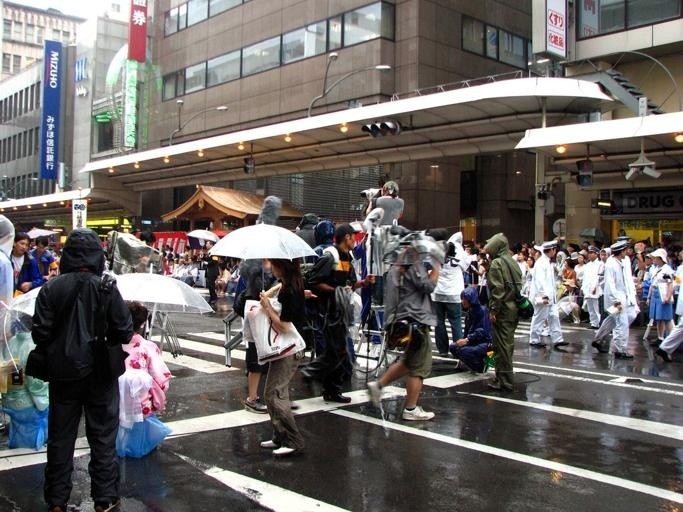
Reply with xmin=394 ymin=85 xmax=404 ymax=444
xmin=353 ymin=221 xmax=389 ymax=389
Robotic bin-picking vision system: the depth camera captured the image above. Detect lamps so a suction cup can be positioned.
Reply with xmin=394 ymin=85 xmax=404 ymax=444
xmin=0 ymin=172 xmax=39 ymax=203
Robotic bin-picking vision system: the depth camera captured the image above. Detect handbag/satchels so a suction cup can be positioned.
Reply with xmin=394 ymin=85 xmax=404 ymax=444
xmin=243 ymin=299 xmax=307 ymax=366
xmin=515 ymin=297 xmax=534 ymax=319
xmin=25 ymin=350 xmax=51 ymax=381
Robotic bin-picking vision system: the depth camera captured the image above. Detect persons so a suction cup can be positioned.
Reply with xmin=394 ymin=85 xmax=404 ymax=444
xmin=11 ymin=228 xmax=240 ymax=304
xmin=0 ymin=214 xmax=16 ymax=314
xmin=0 ymin=305 xmax=51 ymax=448
xmin=365 ymin=181 xmax=404 ymax=225
xmin=235 ymin=215 xmax=522 ymax=420
xmin=24 ymin=228 xmax=133 ymax=511
xmin=259 ymin=258 xmax=306 ymax=455
xmin=118 ymin=302 xmax=168 ymax=450
xmin=510 ymin=237 xmax=682 ymax=370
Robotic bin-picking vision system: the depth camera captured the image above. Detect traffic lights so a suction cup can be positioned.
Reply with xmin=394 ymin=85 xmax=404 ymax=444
xmin=576 ymin=159 xmax=592 ymax=187
xmin=361 ymin=120 xmax=400 ymax=138
xmin=243 ymin=158 xmax=254 ymax=176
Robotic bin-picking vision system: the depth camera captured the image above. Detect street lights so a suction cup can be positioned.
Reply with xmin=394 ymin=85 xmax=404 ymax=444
xmin=0 ymin=175 xmax=38 ymax=202
xmin=67 ymin=186 xmax=91 ymax=229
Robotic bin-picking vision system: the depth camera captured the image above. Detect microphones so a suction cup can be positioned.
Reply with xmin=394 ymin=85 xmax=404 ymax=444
xmin=369 ymin=210 xmax=383 ymax=221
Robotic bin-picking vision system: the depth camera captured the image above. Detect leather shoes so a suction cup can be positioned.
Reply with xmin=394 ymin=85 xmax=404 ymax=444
xmin=529 ymin=326 xmax=672 ymax=363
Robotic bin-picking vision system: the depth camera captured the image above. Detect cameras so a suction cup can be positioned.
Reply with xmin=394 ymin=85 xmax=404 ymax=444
xmin=663 ymin=274 xmax=676 ymax=281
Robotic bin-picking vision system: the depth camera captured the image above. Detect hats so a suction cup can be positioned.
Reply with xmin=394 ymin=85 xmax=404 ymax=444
xmin=535 ymin=236 xmax=668 ymax=264
xmin=336 ymin=223 xmax=360 ymax=235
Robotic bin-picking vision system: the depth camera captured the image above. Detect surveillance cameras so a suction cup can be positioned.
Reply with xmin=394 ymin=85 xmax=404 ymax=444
xmin=625 ymin=168 xmax=639 ymax=182
xmin=643 ymin=166 xmax=662 ymax=179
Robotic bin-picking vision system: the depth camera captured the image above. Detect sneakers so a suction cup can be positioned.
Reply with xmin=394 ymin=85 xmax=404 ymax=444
xmin=48 ymin=506 xmax=67 ymax=512
xmin=245 ymin=353 xmax=513 ymax=455
xmin=94 ymin=497 xmax=121 ymax=512
